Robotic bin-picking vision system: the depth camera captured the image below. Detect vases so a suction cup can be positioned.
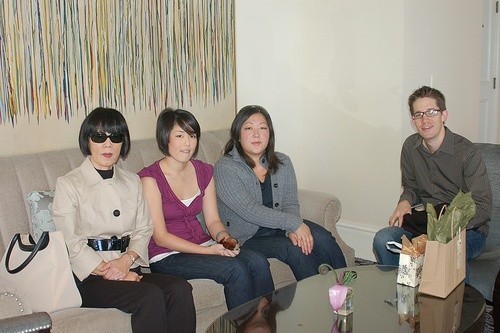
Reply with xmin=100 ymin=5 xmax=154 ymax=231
xmin=334 ymin=289 xmax=354 ymax=315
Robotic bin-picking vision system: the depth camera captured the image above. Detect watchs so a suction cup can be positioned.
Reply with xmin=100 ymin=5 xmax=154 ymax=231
xmin=126 ymin=253 xmax=135 ymax=266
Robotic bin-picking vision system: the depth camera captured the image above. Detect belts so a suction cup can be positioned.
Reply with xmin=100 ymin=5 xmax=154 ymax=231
xmin=87 ymin=235 xmax=131 ymax=253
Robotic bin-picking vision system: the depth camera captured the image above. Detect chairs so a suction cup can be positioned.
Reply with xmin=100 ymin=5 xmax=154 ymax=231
xmin=418 ymin=143 xmax=500 ymax=333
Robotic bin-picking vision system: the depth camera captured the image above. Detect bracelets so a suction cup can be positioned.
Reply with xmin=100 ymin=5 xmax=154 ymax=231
xmin=216 ymin=230 xmax=231 ymax=242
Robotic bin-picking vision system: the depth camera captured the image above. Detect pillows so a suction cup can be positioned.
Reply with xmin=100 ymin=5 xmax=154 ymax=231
xmin=26 ymin=189 xmax=57 ymax=232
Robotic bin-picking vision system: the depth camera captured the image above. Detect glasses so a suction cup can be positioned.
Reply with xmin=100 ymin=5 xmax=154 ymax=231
xmin=412 ymin=108 xmax=444 ymax=121
xmin=86 ymin=131 xmax=125 ymax=144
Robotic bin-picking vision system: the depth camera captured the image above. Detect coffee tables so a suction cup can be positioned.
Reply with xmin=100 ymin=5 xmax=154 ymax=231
xmin=205 ymin=264 xmax=488 ymax=333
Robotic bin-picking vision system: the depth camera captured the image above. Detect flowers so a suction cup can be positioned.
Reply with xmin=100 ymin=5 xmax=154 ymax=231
xmin=318 ymin=265 xmax=358 ymax=312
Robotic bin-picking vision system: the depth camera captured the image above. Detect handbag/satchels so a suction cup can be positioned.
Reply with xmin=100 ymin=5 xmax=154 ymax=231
xmin=402 ymin=202 xmax=451 ymax=239
xmin=418 ymin=204 xmax=467 ymax=299
xmin=396 ymin=284 xmax=419 ymax=330
xmin=1 ymin=231 xmax=83 ymax=321
xmin=419 ymin=279 xmax=465 ymax=333
xmin=385 ymin=240 xmax=425 ymax=287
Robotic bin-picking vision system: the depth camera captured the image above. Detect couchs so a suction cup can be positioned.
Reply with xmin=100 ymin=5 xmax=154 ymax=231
xmin=0 ymin=130 xmax=355 ymax=332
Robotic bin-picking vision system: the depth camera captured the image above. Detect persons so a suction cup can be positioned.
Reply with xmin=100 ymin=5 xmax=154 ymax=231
xmin=52 ymin=107 xmax=197 ymax=333
xmin=136 ymin=106 xmax=275 ymax=312
xmin=372 ymin=84 xmax=494 ymax=266
xmin=224 ymin=282 xmax=298 ymax=333
xmin=212 ymin=107 xmax=346 ymax=282
